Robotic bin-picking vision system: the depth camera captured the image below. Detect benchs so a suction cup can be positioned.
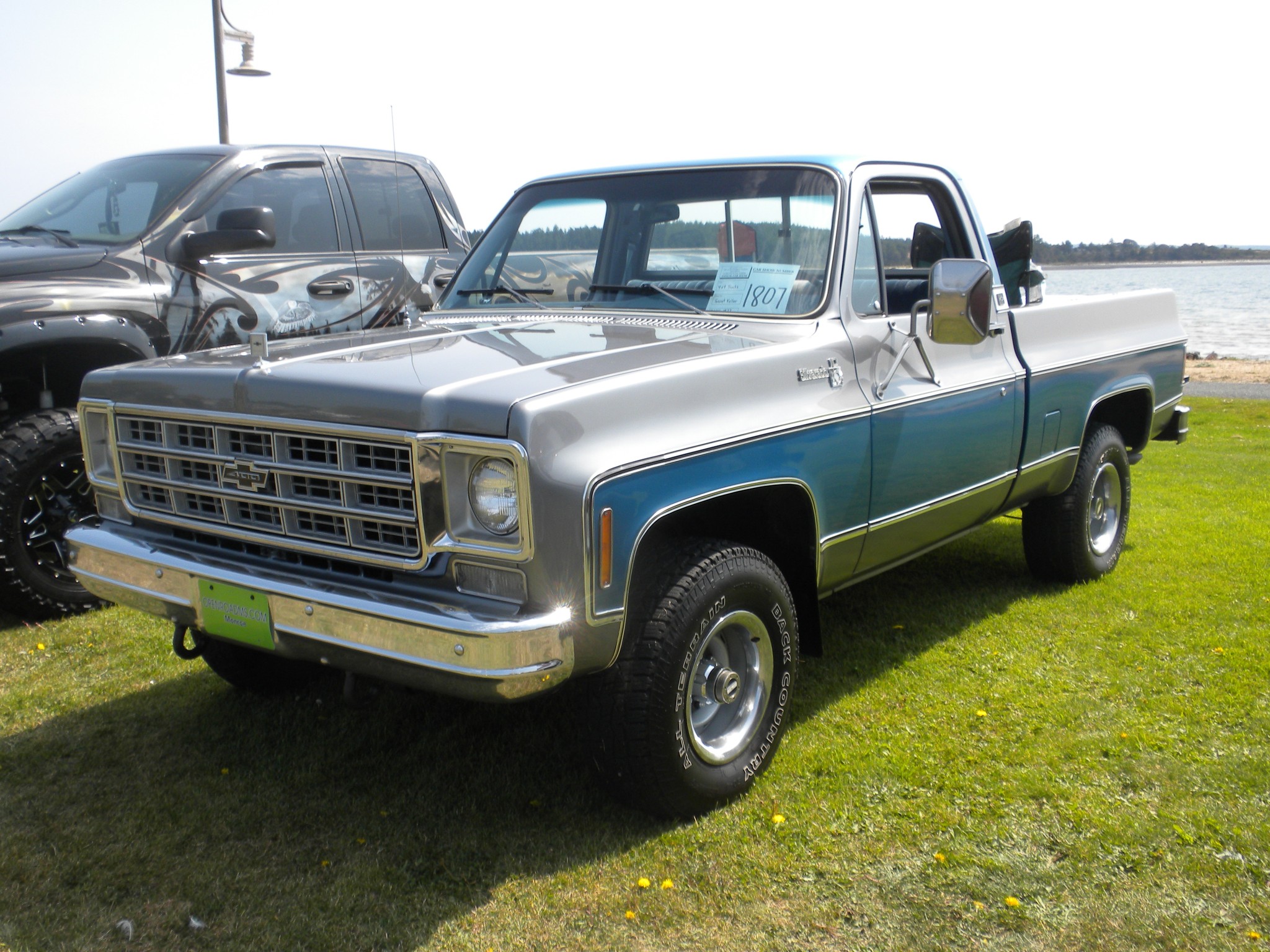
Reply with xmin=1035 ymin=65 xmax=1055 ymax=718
xmin=619 ymin=279 xmax=929 ymax=313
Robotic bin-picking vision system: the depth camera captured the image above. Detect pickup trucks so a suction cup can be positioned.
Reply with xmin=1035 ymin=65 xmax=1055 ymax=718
xmin=0 ymin=150 xmax=724 ymax=620
xmin=65 ymin=162 xmax=1198 ymax=811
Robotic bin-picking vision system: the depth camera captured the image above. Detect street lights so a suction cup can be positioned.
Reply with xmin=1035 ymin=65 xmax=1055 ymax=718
xmin=211 ymin=0 xmax=274 ymax=149
